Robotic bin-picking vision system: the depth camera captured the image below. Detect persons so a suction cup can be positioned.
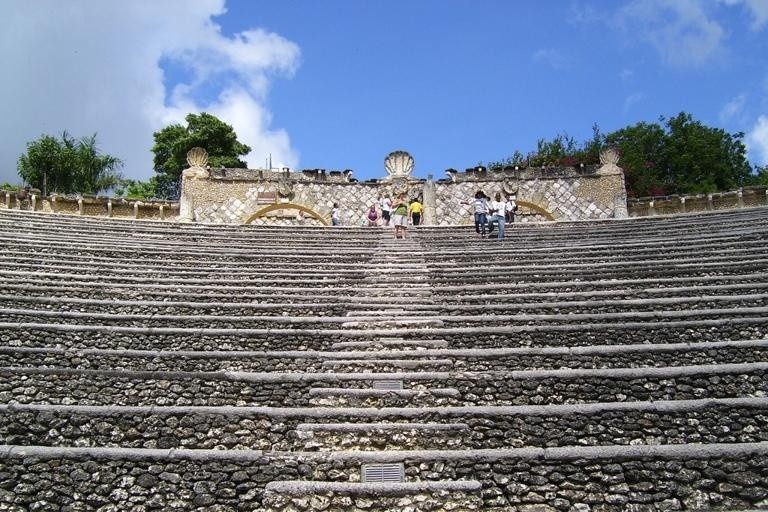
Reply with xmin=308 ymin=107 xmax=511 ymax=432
xmin=366 ymin=205 xmax=380 ymax=226
xmin=460 ymin=191 xmax=492 ymax=238
xmin=410 ymin=198 xmax=423 ymax=226
xmin=504 ymin=194 xmax=517 ymax=223
xmin=485 ymin=197 xmax=491 ymax=223
xmin=487 ymin=192 xmax=506 ymax=247
xmin=392 ymin=192 xmax=408 ymax=240
xmin=295 ymin=210 xmax=305 ymax=225
xmin=330 ymin=203 xmax=339 ymax=226
xmin=382 ymin=194 xmax=392 ymax=226
